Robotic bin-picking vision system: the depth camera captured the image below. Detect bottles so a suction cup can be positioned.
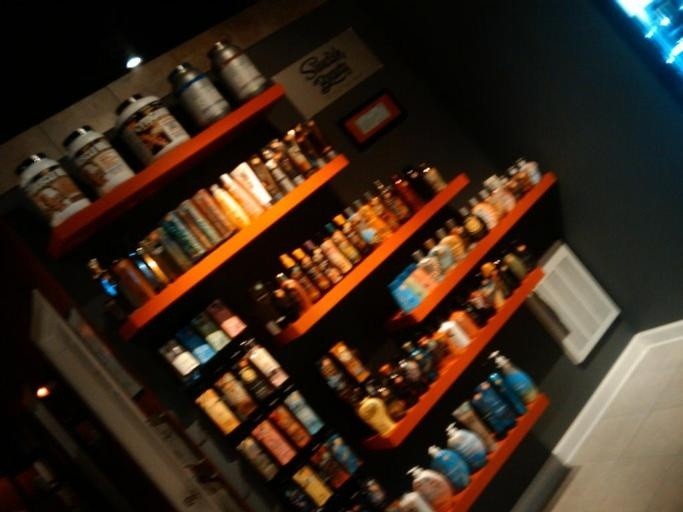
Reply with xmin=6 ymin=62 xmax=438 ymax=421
xmin=205 ymin=37 xmax=274 ymax=99
xmin=370 ymin=337 xmax=444 ymax=422
xmin=165 ymin=62 xmax=232 ymax=127
xmin=59 ymin=122 xmax=137 ymax=196
xmin=13 ymin=149 xmax=92 ymax=230
xmin=114 ymin=91 xmax=192 ymax=167
xmin=274 ymin=161 xmax=551 ymax=314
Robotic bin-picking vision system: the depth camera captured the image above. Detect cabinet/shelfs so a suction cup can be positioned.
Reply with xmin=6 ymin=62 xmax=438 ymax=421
xmin=20 ymin=36 xmax=560 ymax=511
